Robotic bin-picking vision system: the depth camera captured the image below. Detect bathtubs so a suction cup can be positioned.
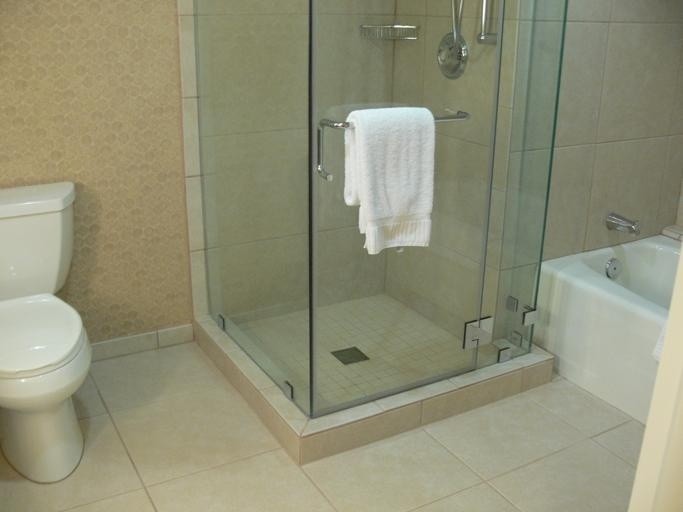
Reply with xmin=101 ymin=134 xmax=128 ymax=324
xmin=530 ymin=234 xmax=681 ymax=427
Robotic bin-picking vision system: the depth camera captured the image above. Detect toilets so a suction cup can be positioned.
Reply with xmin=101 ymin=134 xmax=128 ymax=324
xmin=0 ymin=182 xmax=95 ymax=485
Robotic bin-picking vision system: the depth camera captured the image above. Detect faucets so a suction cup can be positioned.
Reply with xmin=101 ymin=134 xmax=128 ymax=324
xmin=606 ymin=212 xmax=642 ymax=238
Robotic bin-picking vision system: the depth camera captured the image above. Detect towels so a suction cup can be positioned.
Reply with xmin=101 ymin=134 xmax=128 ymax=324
xmin=343 ymin=106 xmax=437 ymax=256
